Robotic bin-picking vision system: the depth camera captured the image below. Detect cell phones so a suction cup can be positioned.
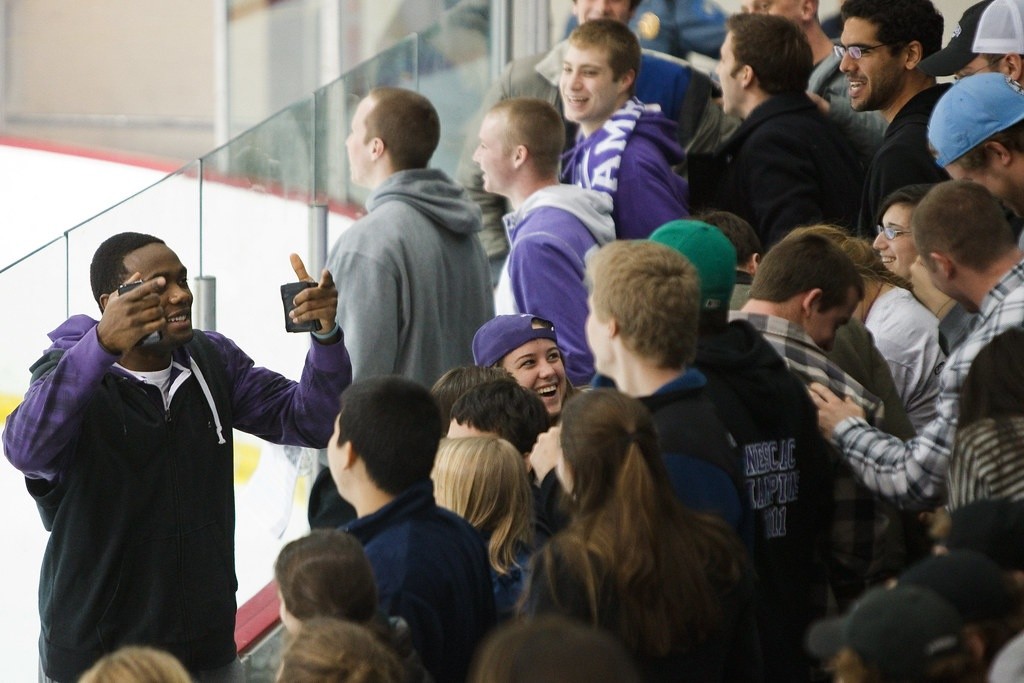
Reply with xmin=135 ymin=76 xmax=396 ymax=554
xmin=117 ymin=280 xmax=160 ymax=345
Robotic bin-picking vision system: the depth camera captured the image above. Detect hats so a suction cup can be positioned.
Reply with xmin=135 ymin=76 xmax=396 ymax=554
xmin=472 ymin=313 xmax=557 ymax=368
xmin=926 ymin=73 xmax=1024 ymax=167
xmin=917 ymin=0 xmax=1024 ymax=77
xmin=808 ymin=586 xmax=983 ymax=683
xmin=648 ymin=219 xmax=738 ymax=312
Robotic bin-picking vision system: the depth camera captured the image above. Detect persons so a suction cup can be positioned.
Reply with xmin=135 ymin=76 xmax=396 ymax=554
xmin=326 ymin=374 xmax=497 ymax=683
xmin=272 ymin=0 xmax=1024 ymax=683
xmin=528 ymin=239 xmax=748 ymax=534
xmin=557 ymin=18 xmax=689 ymax=240
xmin=308 ymin=86 xmax=496 ymax=536
xmin=688 ymin=12 xmax=862 ymax=255
xmin=2 ymin=231 xmax=353 ymax=683
xmin=471 ymin=313 xmax=582 ymax=426
xmin=472 ymin=96 xmax=617 ymax=388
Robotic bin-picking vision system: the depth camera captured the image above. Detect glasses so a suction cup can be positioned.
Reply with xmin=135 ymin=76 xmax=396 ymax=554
xmin=877 ymin=224 xmax=912 ymax=239
xmin=954 ymin=56 xmax=1004 ymax=81
xmin=833 ymin=43 xmax=889 ymax=59
